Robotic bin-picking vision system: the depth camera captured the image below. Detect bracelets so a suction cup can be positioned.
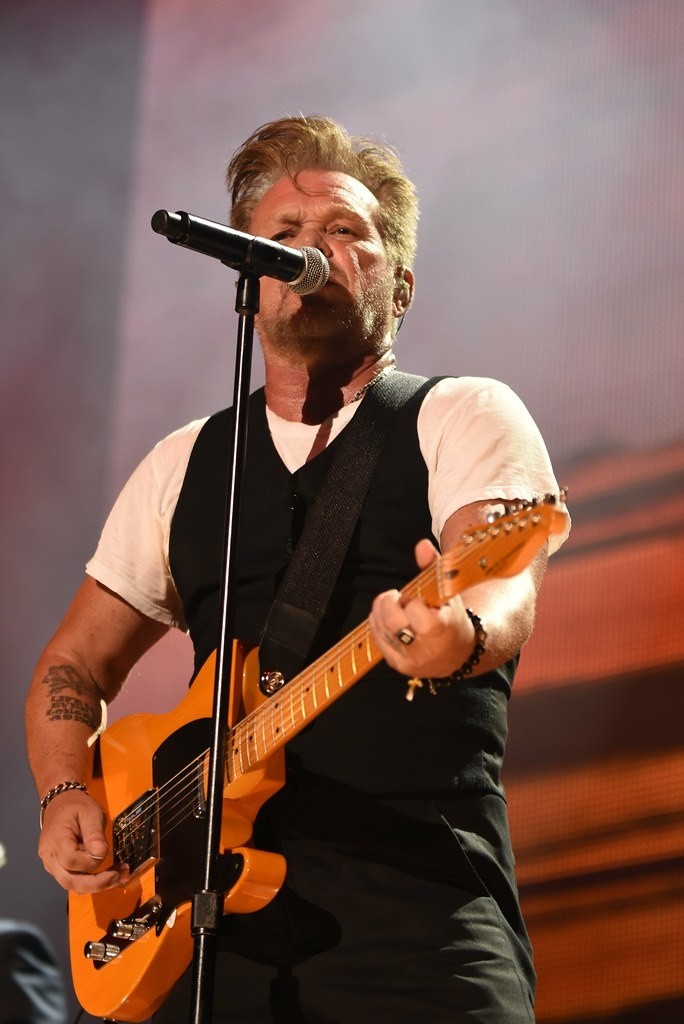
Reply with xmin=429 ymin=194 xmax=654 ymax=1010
xmin=40 ymin=779 xmax=91 ymax=830
xmin=406 ymin=608 xmax=488 ymax=701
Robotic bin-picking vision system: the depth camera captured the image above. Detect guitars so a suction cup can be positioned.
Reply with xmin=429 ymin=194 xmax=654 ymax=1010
xmin=70 ymin=496 xmax=570 ymax=1015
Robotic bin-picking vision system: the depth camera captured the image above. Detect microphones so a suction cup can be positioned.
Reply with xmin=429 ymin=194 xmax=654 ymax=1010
xmin=151 ymin=209 xmax=329 ymax=295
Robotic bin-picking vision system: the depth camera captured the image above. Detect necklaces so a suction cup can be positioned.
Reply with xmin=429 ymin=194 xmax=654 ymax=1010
xmin=344 ymin=364 xmax=396 ymax=407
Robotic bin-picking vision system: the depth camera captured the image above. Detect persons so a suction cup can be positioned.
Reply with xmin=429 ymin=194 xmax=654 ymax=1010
xmin=0 ymin=844 xmax=69 ymax=1024
xmin=25 ymin=112 xmax=572 ymax=1024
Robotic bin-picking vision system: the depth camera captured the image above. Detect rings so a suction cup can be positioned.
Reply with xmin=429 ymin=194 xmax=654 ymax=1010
xmin=397 ymin=625 xmax=417 ymax=646
